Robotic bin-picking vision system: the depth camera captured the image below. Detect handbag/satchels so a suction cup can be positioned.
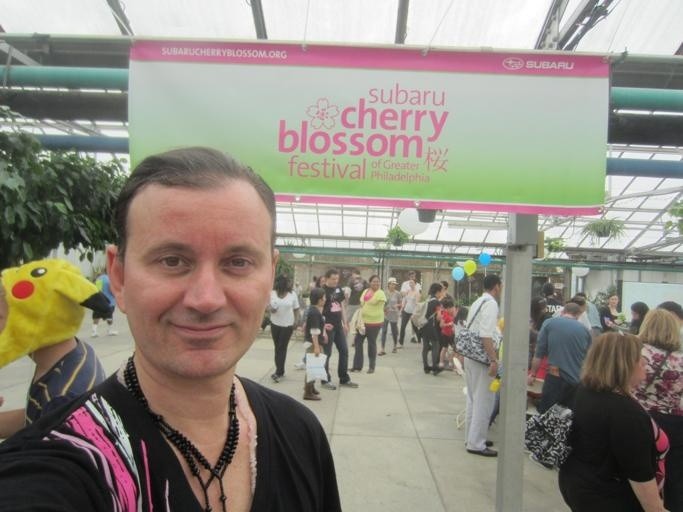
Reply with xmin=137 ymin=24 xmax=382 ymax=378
xmin=524 ymin=389 xmax=575 ymax=471
xmin=454 ymin=298 xmax=503 ymax=366
xmin=304 ymin=353 xmax=328 ymax=382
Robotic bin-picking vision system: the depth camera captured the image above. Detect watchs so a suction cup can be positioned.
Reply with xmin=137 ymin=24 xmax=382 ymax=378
xmin=530 ymin=374 xmax=536 ymax=377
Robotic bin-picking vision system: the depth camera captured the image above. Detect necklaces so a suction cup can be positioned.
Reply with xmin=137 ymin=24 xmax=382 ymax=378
xmin=123 ymin=354 xmax=241 ymax=512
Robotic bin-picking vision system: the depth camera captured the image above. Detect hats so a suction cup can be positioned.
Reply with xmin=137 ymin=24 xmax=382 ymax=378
xmin=387 ymin=277 xmax=399 ymax=285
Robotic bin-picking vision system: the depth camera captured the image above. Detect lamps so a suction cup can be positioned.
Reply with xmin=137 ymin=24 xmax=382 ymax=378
xmin=398 ymin=207 xmax=429 ymax=235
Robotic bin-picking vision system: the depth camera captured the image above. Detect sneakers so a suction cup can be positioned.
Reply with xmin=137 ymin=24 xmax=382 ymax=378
xmin=321 ymin=382 xmax=337 ymax=390
xmin=433 ymin=367 xmax=444 ymax=376
xmin=91 ymin=329 xmax=98 ymax=337
xmin=271 ymin=372 xmax=284 ymax=381
xmin=424 ymin=366 xmax=432 ymax=375
xmin=107 ymin=329 xmax=117 ymax=336
xmin=340 ymin=379 xmax=359 ymax=388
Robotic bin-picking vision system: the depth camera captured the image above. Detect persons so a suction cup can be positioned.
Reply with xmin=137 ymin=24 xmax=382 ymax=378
xmin=629 ymin=307 xmax=683 ymax=512
xmin=630 ymin=302 xmax=649 ymax=335
xmin=489 ymin=316 xmax=504 ymax=431
xmin=600 ymin=294 xmax=630 ymax=333
xmin=347 ymin=274 xmax=387 ymax=374
xmin=464 ymin=274 xmax=501 ymax=457
xmin=91 ymin=267 xmax=119 ymax=337
xmin=265 ymin=275 xmax=300 ymax=385
xmin=377 ymin=277 xmax=402 ymax=355
xmin=418 ymin=283 xmax=444 ymax=375
xmin=539 ymin=283 xmax=564 ymax=316
xmin=558 ymin=331 xmax=670 ymax=512
xmin=552 ymin=296 xmax=594 ymax=336
xmin=296 ymin=283 xmax=304 ymax=298
xmin=0 ymin=147 xmax=341 ymax=512
xmin=345 ymin=269 xmax=371 ymax=347
xmin=302 ymin=288 xmax=334 ymax=400
xmin=439 ymin=298 xmax=456 ymax=371
xmin=528 ymin=303 xmax=591 ymax=416
xmin=316 ymin=275 xmax=326 ymax=289
xmin=0 ymin=259 xmax=106 ymax=440
xmin=575 ymin=293 xmax=603 ymax=337
xmin=310 ymin=275 xmax=318 ymax=290
xmin=453 ymin=307 xmax=469 ymax=375
xmin=529 ymin=299 xmax=548 ymax=324
xmin=440 ymin=281 xmax=453 ymax=301
xmin=322 ymin=269 xmax=358 ymax=390
xmin=658 ymin=302 xmax=682 ymax=321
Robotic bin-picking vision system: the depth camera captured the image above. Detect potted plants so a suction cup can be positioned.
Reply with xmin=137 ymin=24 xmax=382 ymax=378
xmin=386 ymin=224 xmax=415 ymax=248
xmin=417 ymin=208 xmax=443 ymax=223
xmin=545 ymin=235 xmax=567 ymax=252
xmin=580 ymin=218 xmax=630 ymax=244
xmin=663 ymin=200 xmax=683 ymax=235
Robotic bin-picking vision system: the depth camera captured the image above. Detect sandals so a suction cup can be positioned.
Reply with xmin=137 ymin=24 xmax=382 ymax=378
xmin=391 ymin=347 xmax=397 ymax=353
xmin=377 ymin=351 xmax=386 ymax=357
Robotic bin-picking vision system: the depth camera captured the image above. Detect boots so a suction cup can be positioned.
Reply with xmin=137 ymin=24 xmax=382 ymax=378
xmin=303 ymin=381 xmax=322 ymax=400
xmin=309 ymin=381 xmax=320 ymax=395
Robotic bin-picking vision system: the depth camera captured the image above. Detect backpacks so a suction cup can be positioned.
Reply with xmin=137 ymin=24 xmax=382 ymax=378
xmin=411 ymin=298 xmax=441 ymax=330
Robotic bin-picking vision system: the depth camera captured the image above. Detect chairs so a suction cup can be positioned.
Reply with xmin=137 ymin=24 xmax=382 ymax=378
xmin=525 ymin=376 xmax=545 ymax=418
xmin=451 ymin=356 xmax=497 ymax=429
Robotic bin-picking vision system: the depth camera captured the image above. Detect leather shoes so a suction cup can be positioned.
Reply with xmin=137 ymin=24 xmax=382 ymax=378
xmin=485 ymin=440 xmax=494 ymax=447
xmin=365 ymin=366 xmax=375 ymax=374
xmin=468 ymin=446 xmax=496 ymax=459
xmin=348 ymin=367 xmax=363 ymax=372
